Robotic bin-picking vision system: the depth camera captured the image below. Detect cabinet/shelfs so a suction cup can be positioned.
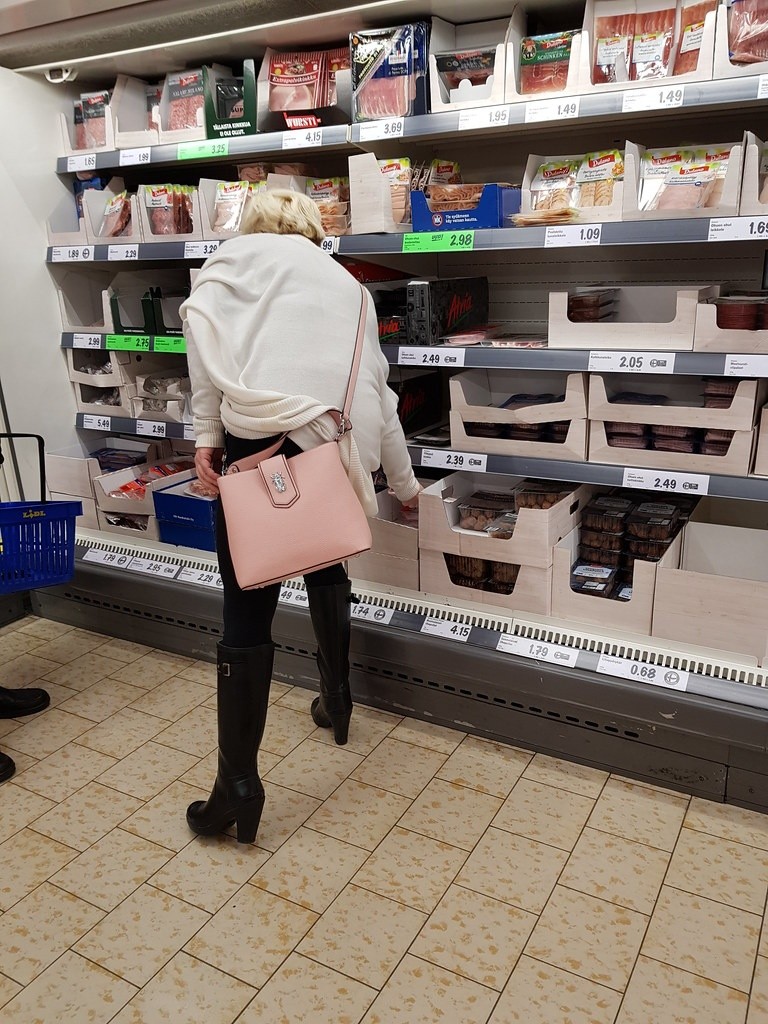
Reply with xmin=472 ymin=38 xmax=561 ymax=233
xmin=51 ymin=0 xmax=768 ymax=818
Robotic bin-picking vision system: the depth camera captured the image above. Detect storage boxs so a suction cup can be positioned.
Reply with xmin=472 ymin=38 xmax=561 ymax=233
xmin=65 ymin=1 xmax=768 ymax=667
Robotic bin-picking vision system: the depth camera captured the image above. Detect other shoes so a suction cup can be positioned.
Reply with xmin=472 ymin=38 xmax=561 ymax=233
xmin=0 ymin=681 xmax=50 ymax=719
xmin=0 ymin=750 xmax=17 ymax=783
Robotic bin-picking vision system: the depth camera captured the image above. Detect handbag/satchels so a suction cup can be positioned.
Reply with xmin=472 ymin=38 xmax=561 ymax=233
xmin=218 ymin=439 xmax=376 ymax=592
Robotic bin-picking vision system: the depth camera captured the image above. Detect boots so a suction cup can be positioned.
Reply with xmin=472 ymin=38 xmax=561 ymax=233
xmin=187 ymin=641 xmax=279 ymax=845
xmin=310 ymin=581 xmax=357 ymax=745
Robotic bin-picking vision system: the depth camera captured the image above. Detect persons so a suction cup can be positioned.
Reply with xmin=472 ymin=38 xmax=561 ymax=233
xmin=179 ymin=189 xmax=424 ymax=843
